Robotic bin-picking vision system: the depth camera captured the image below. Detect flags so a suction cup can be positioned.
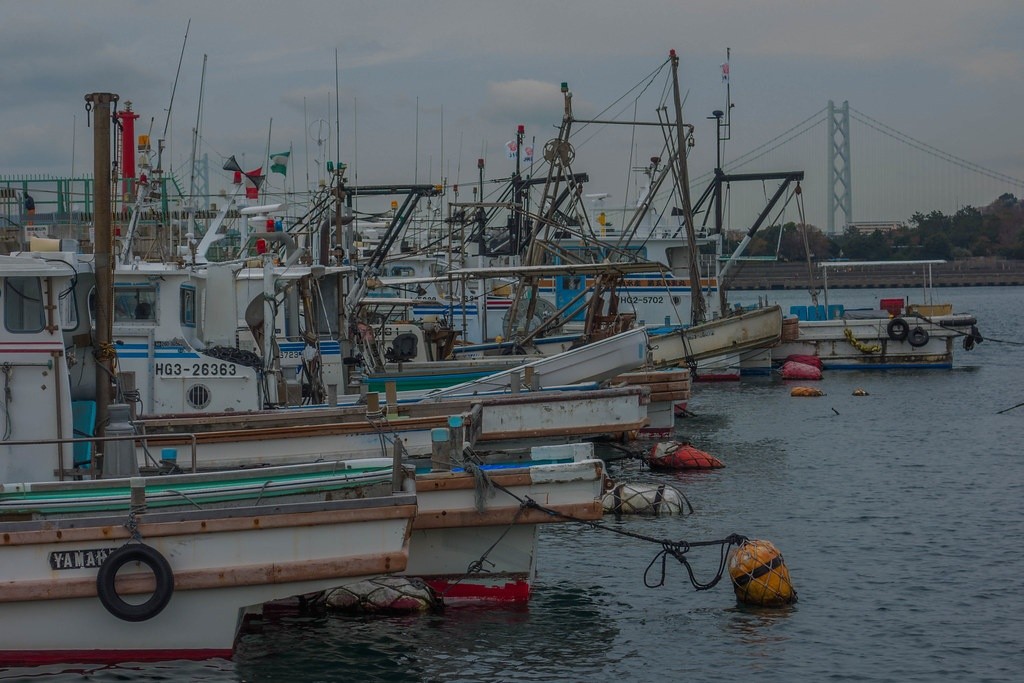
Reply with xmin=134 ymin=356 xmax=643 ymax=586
xmin=269 ymin=152 xmax=290 ymax=176
xmin=245 ymin=167 xmax=262 ymax=200
xmin=523 ymin=143 xmax=534 ymax=163
xmin=721 ymin=64 xmax=729 ymax=82
xmin=506 ymin=138 xmax=517 ymax=159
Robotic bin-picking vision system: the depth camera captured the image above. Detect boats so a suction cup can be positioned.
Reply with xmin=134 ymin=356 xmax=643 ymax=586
xmin=0 ymin=17 xmax=984 ymax=667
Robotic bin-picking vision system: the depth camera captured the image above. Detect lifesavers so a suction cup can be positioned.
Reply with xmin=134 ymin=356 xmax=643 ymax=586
xmin=334 ymin=246 xmax=345 ymax=259
xmin=887 ymin=318 xmax=930 ymax=347
xmin=95 ymin=542 xmax=175 ymax=624
